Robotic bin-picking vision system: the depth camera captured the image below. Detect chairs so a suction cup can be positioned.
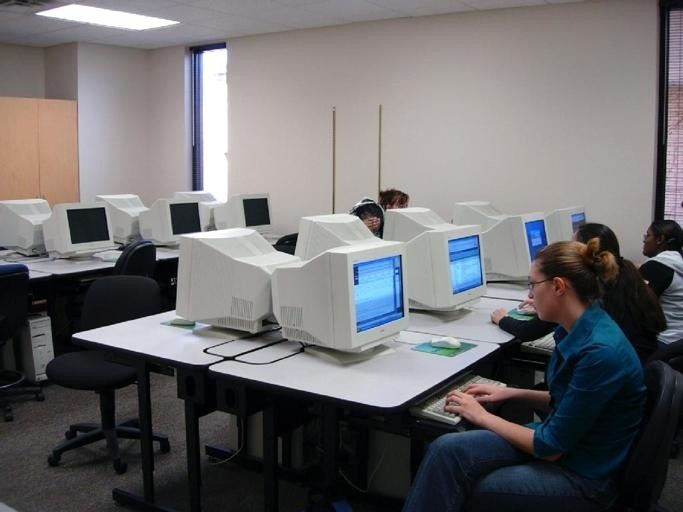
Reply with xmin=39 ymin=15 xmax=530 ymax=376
xmin=1 ymin=264 xmax=44 ymax=423
xmin=276 ymin=233 xmax=299 ymax=245
xmin=111 ymin=242 xmax=158 ymax=278
xmin=590 ymin=360 xmax=682 ymax=511
xmin=272 ymin=243 xmax=298 ymax=256
xmin=650 ymin=338 xmax=682 ymax=372
xmin=45 ymin=276 xmax=171 ymax=474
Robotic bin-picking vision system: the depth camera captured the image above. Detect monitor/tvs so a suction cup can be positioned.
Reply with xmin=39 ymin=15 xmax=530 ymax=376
xmin=545 ymin=206 xmax=585 ymax=244
xmin=481 ymin=213 xmax=549 ymax=290
xmin=0 ymin=191 xmax=273 ymax=258
xmin=271 ymin=241 xmax=410 ymax=366
xmin=453 ymin=200 xmax=507 ymax=232
xmin=382 ymin=207 xmax=457 ymax=242
xmin=404 ymin=225 xmax=487 ymax=321
xmin=175 ymin=228 xmax=301 ymax=340
xmin=294 ymin=214 xmax=385 ymax=261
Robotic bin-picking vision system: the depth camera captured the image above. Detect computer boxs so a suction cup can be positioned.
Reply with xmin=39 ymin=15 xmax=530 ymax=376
xmin=231 ymin=411 xmax=323 ymax=470
xmin=0 ymin=314 xmax=54 ymax=383
xmin=366 ymin=429 xmax=412 ymax=500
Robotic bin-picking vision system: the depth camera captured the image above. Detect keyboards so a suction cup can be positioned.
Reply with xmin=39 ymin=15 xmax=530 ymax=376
xmin=521 ymin=331 xmax=556 ymax=356
xmin=409 ymin=375 xmax=507 ymax=426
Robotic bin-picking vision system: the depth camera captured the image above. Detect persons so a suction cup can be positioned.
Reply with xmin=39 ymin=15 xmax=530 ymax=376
xmin=380 ymin=190 xmax=408 ymax=211
xmin=492 ymin=223 xmax=668 ymax=423
xmin=638 ymin=220 xmax=683 ymax=362
xmin=349 ymin=197 xmax=384 ymax=239
xmin=401 ymin=238 xmax=647 ymax=511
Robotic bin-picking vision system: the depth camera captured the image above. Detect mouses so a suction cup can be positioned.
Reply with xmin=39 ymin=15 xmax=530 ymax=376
xmin=431 ymin=336 xmax=460 ymax=349
xmin=516 ymin=302 xmax=528 ymax=314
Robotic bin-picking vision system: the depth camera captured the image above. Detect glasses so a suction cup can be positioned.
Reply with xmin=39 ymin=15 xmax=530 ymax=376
xmin=528 ymin=279 xmax=552 ymax=290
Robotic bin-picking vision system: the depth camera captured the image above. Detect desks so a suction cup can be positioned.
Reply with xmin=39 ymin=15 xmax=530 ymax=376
xmin=71 ymin=280 xmax=538 ymax=511
xmin=1 ymin=240 xmax=180 ymax=386
